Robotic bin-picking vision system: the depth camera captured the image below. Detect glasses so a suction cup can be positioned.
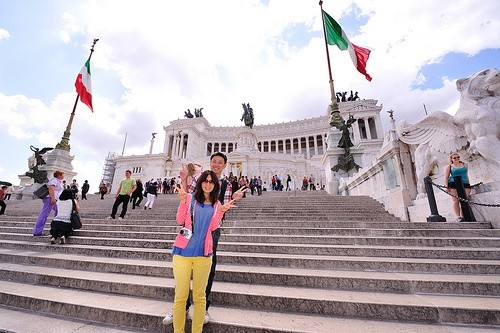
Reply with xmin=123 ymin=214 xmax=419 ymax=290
xmin=453 ymin=157 xmax=459 ymax=159
xmin=204 ymin=179 xmax=214 ymax=183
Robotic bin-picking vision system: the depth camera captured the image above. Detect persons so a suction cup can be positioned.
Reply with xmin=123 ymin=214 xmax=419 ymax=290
xmin=173 ymin=170 xmax=239 ymax=333
xmin=0 ymin=186 xmax=8 ymax=215
xmin=107 ymin=170 xmax=137 ymax=220
xmin=303 ymin=174 xmax=321 ymax=190
xmin=223 ymin=172 xmax=292 ymax=204
xmin=63 ymin=179 xmax=79 ymax=200
xmin=444 ymin=154 xmax=471 ymax=222
xmin=132 ymin=178 xmax=144 ymax=209
xmin=81 ymin=180 xmax=89 ymax=200
xmin=4 ymin=183 xmax=15 ymax=200
xmin=163 ymin=153 xmax=249 ymax=325
xmin=33 ymin=170 xmax=64 ymax=236
xmin=49 ymin=189 xmax=79 ymax=244
xmin=144 ymin=182 xmax=157 ymax=210
xmin=99 ymin=180 xmax=112 ymax=200
xmin=144 ymin=175 xmax=181 ymax=194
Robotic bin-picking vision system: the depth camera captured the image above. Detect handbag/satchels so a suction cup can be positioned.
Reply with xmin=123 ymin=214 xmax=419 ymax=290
xmin=70 ymin=199 xmax=82 ymax=230
xmin=309 ymin=177 xmax=311 ymax=182
xmin=100 ymin=187 xmax=103 ymax=192
xmin=130 ymin=194 xmax=133 ymax=203
xmin=34 ymin=178 xmax=58 ymax=199
xmin=144 ymin=192 xmax=147 ymax=197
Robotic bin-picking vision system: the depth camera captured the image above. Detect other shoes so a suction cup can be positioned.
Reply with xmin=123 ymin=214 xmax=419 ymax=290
xmin=107 ymin=217 xmax=113 ymax=219
xmin=50 ymin=238 xmax=56 ymax=244
xmin=204 ymin=311 xmax=210 ymax=324
xmin=148 ymin=208 xmax=151 ymax=209
xmin=144 ymin=206 xmax=146 ymax=210
xmin=118 ymin=217 xmax=122 ymax=220
xmin=455 ymin=217 xmax=463 ymax=222
xmin=162 ymin=308 xmax=190 ymax=325
xmin=59 ymin=236 xmax=65 ymax=245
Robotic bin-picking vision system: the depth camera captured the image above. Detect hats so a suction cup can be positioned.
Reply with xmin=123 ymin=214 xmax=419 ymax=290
xmin=85 ymin=180 xmax=88 ymax=182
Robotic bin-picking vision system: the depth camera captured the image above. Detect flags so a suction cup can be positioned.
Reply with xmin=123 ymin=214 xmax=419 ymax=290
xmin=322 ymin=9 xmax=372 ymax=82
xmin=75 ymin=56 xmax=93 ymax=112
